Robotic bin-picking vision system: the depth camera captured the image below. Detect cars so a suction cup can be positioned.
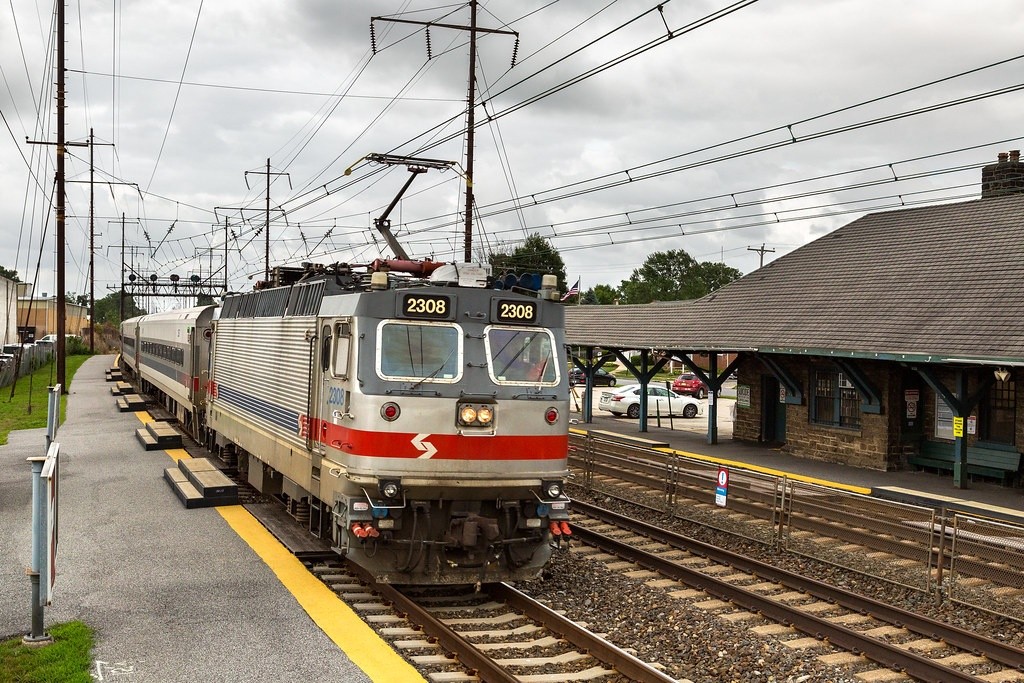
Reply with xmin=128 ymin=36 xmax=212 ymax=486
xmin=598 ymin=384 xmax=705 ymax=419
xmin=568 ymin=366 xmax=617 ymax=387
xmin=672 ymin=372 xmax=723 ymax=398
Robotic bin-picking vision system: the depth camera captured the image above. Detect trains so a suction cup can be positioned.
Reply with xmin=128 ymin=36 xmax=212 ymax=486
xmin=119 ymin=259 xmax=572 ymax=593
